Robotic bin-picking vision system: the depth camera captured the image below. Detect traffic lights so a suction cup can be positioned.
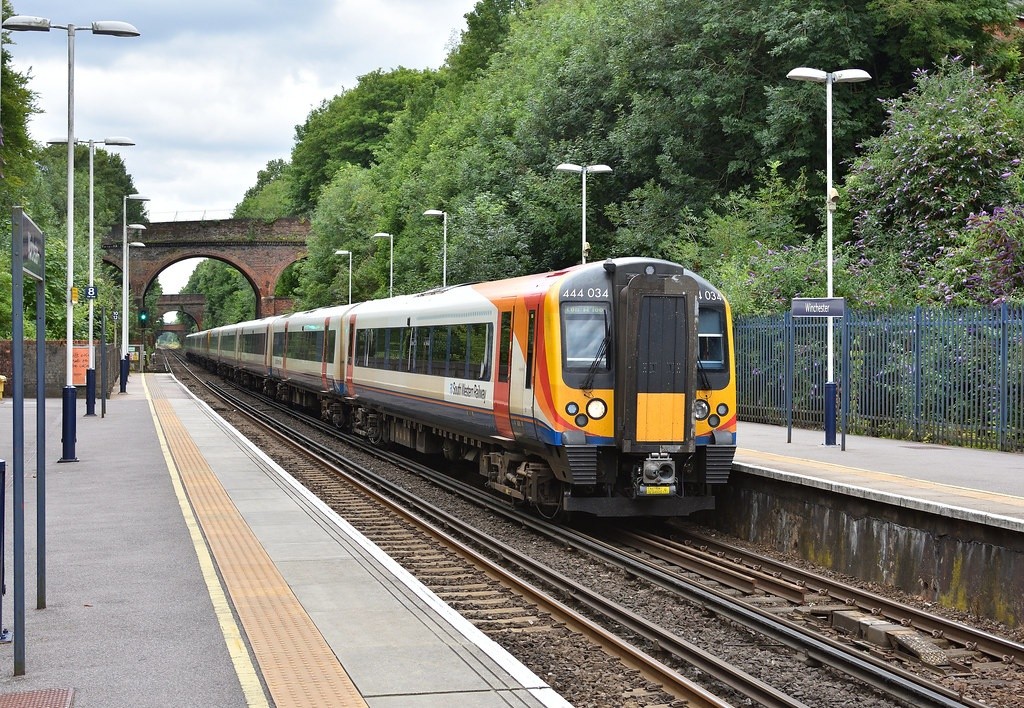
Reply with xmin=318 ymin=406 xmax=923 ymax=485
xmin=138 ymin=307 xmax=149 ymax=328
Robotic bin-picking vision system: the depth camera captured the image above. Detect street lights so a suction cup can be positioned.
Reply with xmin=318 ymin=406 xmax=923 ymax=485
xmin=422 ymin=209 xmax=446 ymax=289
xmin=334 ymin=250 xmax=353 ymax=305
xmin=554 ymin=162 xmax=614 ymax=264
xmin=119 ymin=193 xmax=151 ymax=394
xmin=2 ymin=13 xmax=141 ymax=467
xmin=45 ymin=137 xmax=135 ymax=414
xmin=787 ymin=66 xmax=872 ymax=447
xmin=372 ymin=232 xmax=393 ymax=297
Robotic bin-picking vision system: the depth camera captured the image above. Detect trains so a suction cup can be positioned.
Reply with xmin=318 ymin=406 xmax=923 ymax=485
xmin=184 ymin=255 xmax=737 ymax=525
